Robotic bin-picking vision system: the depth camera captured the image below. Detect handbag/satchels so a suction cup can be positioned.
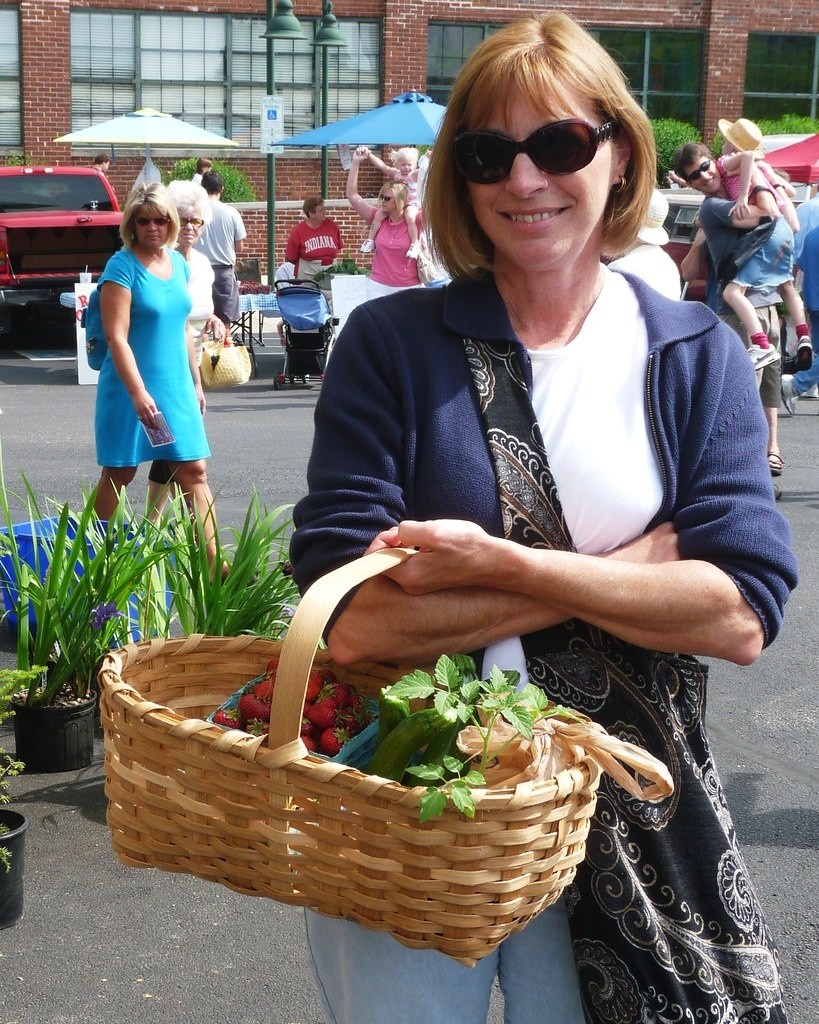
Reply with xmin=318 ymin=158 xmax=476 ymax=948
xmin=79 ymin=288 xmax=108 ymax=371
xmin=520 ymin=623 xmax=787 ymax=1024
xmin=199 ymin=335 xmax=251 ymax=390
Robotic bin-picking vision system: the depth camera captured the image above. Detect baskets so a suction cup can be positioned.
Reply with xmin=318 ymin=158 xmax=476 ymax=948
xmin=97 ymin=546 xmax=607 ymax=968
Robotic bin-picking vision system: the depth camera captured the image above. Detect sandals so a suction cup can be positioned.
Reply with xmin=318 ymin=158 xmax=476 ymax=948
xmin=766 ymin=453 xmax=784 ymax=476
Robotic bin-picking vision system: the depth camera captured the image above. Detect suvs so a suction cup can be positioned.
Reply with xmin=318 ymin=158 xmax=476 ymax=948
xmin=0 ymin=166 xmax=123 ymax=348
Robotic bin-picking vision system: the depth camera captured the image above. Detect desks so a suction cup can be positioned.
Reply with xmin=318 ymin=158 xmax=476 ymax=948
xmin=234 ymin=294 xmax=282 ymax=377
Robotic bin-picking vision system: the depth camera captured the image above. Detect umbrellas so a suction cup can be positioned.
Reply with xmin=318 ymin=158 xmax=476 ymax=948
xmin=268 ymin=84 xmax=448 ymax=146
xmin=53 ymin=106 xmax=238 ymax=185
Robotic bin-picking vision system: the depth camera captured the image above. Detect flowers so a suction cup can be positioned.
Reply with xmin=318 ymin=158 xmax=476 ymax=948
xmin=2 ymin=467 xmax=302 ymax=708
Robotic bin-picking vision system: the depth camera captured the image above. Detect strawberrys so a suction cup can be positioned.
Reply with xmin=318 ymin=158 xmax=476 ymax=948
xmin=212 ymin=656 xmax=376 ymax=757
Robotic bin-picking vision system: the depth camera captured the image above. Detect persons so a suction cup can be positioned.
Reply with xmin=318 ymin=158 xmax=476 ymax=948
xmin=607 ymin=188 xmax=681 ymax=300
xmin=192 ymin=170 xmax=247 ymax=345
xmin=93 ymin=154 xmax=109 ymax=172
xmin=275 ymin=259 xmax=296 ymax=290
xmin=290 ymin=5 xmax=799 ymax=1024
xmin=144 ymin=178 xmax=227 ymax=525
xmin=666 ymin=170 xmax=687 ymax=188
xmin=277 ymin=319 xmax=286 ymax=346
xmin=286 ymin=196 xmax=344 ymax=290
xmin=93 ymin=182 xmax=233 ymax=587
xmin=191 ymin=157 xmax=212 ymax=184
xmin=672 ymin=113 xmax=819 ymax=476
xmin=345 ymin=145 xmax=452 ymax=300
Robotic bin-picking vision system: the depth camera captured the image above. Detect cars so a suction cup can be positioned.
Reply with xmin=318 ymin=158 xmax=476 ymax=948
xmin=600 ymin=193 xmax=708 ymax=304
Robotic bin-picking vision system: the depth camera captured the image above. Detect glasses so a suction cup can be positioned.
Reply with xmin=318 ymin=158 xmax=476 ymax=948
xmin=180 ymin=217 xmax=204 ymax=227
xmin=452 ymin=117 xmax=618 ymax=183
xmin=686 ymin=159 xmax=712 ymax=181
xmin=380 ymin=194 xmax=396 ymax=202
xmin=134 ymin=217 xmax=170 ymax=226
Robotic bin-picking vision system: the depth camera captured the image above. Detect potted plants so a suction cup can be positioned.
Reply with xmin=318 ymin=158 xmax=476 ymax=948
xmin=313 ymin=257 xmax=370 ymax=291
xmin=0 ymin=748 xmax=28 ymax=928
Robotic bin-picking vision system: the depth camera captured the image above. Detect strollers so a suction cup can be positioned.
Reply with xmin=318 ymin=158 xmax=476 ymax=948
xmin=273 ymin=279 xmax=339 ymax=389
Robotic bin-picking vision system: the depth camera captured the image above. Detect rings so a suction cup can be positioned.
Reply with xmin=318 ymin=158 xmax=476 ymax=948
xmin=137 ymin=415 xmax=142 ymax=420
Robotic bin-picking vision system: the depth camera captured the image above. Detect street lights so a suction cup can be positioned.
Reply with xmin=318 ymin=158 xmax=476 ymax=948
xmin=309 ymin=0 xmax=348 ymax=200
xmin=258 ymin=0 xmax=310 ymax=291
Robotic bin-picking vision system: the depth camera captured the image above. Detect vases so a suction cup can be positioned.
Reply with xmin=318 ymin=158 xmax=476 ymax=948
xmin=12 ymin=687 xmax=97 ymax=772
xmin=46 ymin=660 xmax=104 ymax=738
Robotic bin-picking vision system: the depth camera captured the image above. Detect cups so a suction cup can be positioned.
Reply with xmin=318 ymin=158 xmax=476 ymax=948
xmin=80 ymin=273 xmax=92 ymax=283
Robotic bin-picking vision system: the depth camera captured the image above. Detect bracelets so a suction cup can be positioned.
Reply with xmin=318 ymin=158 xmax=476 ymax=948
xmin=772 ymin=183 xmax=785 ymax=188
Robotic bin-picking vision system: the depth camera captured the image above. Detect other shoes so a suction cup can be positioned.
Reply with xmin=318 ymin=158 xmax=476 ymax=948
xmin=773 ymin=483 xmax=782 ymax=499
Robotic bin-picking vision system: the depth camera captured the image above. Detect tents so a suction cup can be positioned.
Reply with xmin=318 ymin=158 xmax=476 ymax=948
xmin=762 ymin=133 xmax=819 ymax=201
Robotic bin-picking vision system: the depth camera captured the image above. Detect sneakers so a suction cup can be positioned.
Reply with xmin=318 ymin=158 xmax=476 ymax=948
xmin=806 ymin=384 xmax=818 ymax=398
xmin=796 ymin=335 xmax=813 ymax=371
xmin=780 ymin=374 xmax=800 ymax=413
xmin=360 ymin=240 xmax=375 ymax=253
xmin=746 ymin=344 xmax=780 ymax=370
xmin=406 ymin=243 xmax=420 ymax=260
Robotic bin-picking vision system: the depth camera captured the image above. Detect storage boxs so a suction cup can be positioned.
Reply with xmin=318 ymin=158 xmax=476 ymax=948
xmin=1 ymin=514 xmax=174 ymax=646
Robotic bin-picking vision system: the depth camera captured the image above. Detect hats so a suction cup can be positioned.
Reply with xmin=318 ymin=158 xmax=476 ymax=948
xmin=718 ymin=118 xmax=765 ymax=160
xmin=635 ymin=190 xmax=668 ymax=245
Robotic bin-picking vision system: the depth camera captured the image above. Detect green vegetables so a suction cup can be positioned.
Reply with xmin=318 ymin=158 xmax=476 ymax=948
xmin=312 ymin=255 xmax=373 ymax=283
